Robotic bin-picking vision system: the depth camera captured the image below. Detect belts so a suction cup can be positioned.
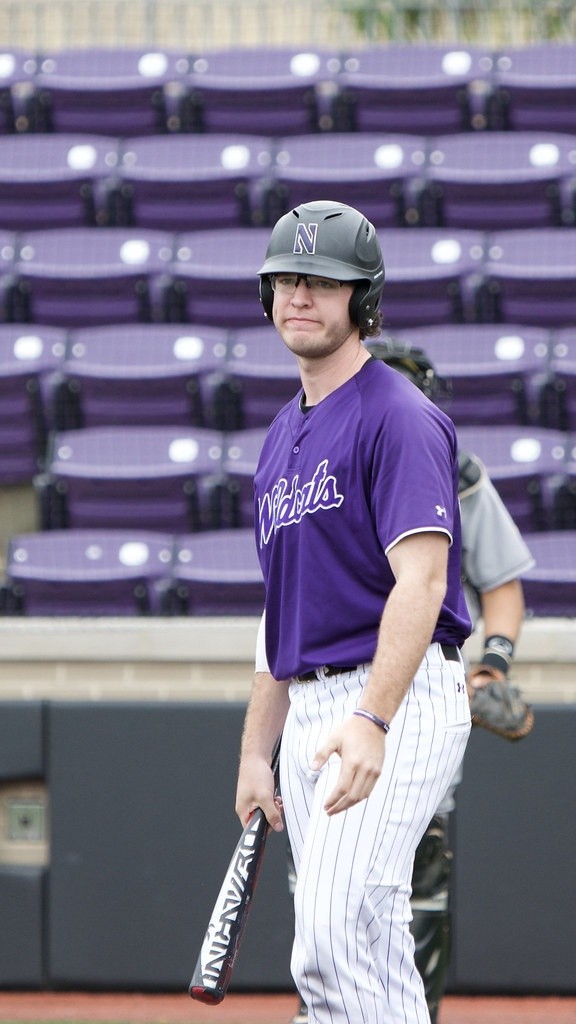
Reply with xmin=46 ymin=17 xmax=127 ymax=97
xmin=292 ymin=644 xmax=462 ymax=684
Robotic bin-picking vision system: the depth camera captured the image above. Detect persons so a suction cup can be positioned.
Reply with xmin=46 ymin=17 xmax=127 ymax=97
xmin=235 ymin=202 xmax=474 ymax=1024
xmin=369 ymin=344 xmax=538 ymax=1023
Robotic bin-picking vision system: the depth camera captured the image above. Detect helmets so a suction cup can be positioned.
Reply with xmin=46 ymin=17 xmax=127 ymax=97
xmin=365 ymin=338 xmax=436 ymax=404
xmin=257 ymin=200 xmax=385 ymax=329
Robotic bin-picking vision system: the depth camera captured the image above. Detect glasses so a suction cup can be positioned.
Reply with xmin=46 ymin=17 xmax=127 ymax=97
xmin=268 ymin=273 xmax=357 ymax=296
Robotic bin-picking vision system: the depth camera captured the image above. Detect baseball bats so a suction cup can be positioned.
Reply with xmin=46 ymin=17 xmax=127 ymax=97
xmin=186 ymin=749 xmax=281 ymax=1008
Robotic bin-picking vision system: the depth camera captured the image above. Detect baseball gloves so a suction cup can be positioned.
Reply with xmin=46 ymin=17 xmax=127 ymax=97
xmin=459 ymin=664 xmax=537 ymax=743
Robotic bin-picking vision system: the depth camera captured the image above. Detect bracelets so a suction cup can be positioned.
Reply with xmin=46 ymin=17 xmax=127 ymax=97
xmin=482 ymin=635 xmax=514 ymax=675
xmin=354 ymin=709 xmax=390 ymax=734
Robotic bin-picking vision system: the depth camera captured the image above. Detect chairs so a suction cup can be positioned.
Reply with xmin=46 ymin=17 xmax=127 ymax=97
xmin=0 ymin=44 xmax=576 ymax=618
